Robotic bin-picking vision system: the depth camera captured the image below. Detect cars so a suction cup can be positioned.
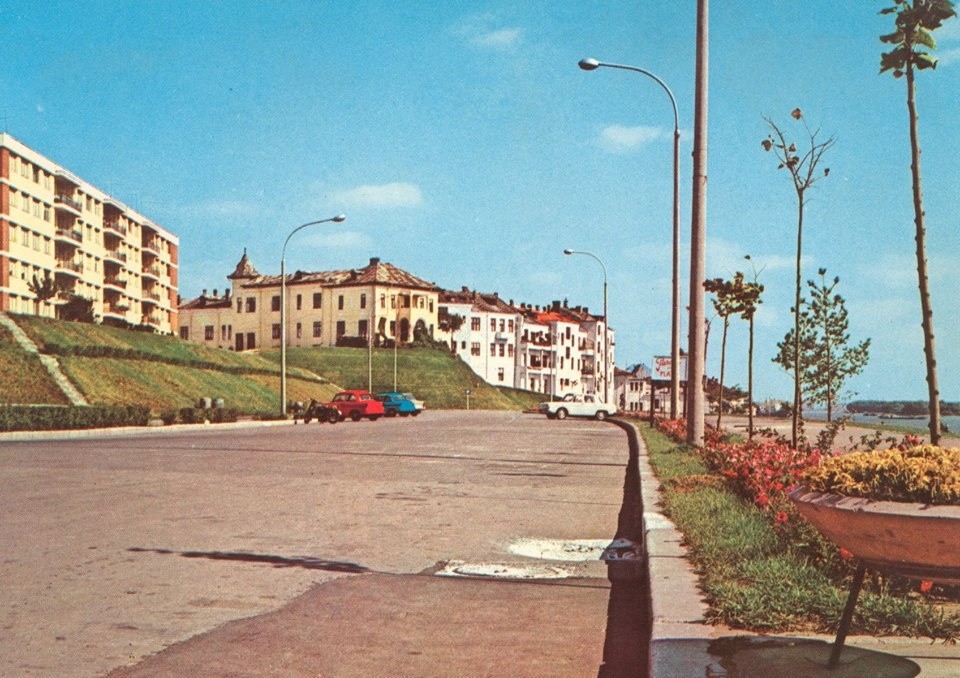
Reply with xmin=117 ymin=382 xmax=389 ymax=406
xmin=325 ymin=390 xmax=426 ymax=421
xmin=538 ymin=393 xmax=618 ymax=420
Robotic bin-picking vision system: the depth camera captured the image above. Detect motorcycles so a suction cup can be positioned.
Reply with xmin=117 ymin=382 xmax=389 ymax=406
xmin=302 ymin=395 xmax=343 ymax=424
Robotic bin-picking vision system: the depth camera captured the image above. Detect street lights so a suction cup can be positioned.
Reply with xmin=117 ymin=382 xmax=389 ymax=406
xmin=578 ymin=57 xmax=678 ymax=421
xmin=562 ymin=248 xmax=609 ymax=402
xmin=280 ymin=214 xmax=346 ymax=417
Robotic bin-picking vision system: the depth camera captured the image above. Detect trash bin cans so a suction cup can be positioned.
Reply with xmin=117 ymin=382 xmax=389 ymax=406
xmin=213 ymin=398 xmax=225 ymax=408
xmin=295 ymin=401 xmax=303 ymax=413
xmin=201 ymin=397 xmax=212 ymax=409
